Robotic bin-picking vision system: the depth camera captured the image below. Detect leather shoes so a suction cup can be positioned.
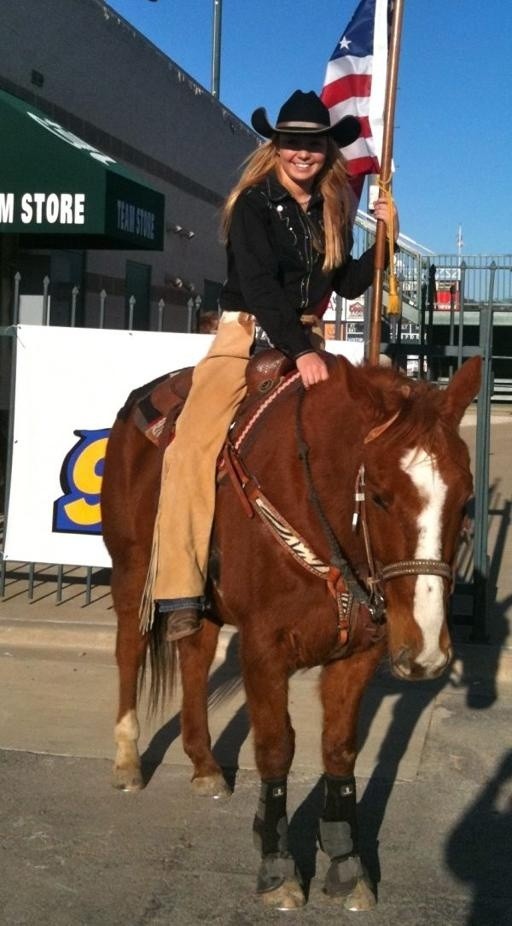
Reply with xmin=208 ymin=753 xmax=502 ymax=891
xmin=167 ymin=607 xmax=203 ymax=640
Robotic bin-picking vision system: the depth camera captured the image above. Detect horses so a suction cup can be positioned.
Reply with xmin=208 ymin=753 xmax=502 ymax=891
xmin=101 ymin=354 xmax=482 ymax=912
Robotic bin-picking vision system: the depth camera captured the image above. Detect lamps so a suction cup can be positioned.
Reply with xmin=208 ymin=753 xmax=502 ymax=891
xmin=167 ymin=224 xmax=195 ymax=239
xmin=167 ymin=275 xmax=182 ymax=288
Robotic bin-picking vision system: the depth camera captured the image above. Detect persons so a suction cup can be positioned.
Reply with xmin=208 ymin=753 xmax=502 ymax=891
xmin=146 ymin=89 xmax=401 ymax=645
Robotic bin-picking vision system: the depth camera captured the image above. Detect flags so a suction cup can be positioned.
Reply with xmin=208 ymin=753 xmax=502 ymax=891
xmin=320 ymin=1 xmax=397 ymax=178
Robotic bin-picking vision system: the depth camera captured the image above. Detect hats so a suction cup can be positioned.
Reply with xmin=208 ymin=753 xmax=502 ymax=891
xmin=252 ymin=89 xmax=362 ymax=150
